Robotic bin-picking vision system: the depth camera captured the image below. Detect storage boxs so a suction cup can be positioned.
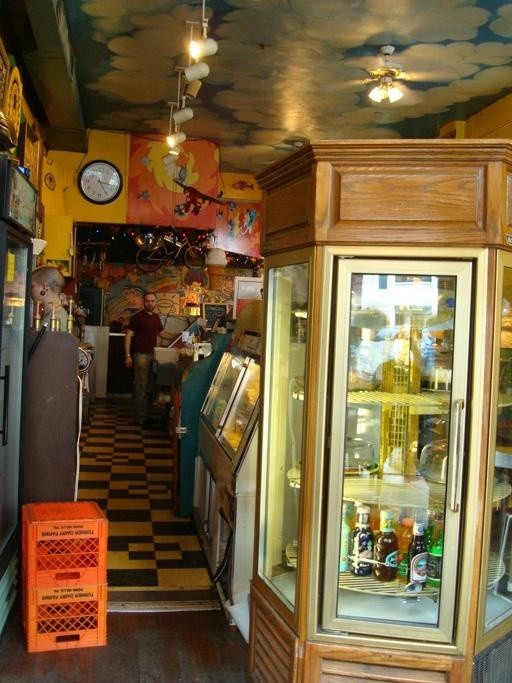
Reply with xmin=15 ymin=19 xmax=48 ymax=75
xmin=154 ymin=347 xmax=180 ymax=373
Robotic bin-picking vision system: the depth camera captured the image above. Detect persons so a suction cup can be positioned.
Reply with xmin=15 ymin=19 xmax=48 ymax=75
xmin=31 ymin=265 xmax=76 ymax=336
xmin=124 ymin=290 xmax=180 ymax=424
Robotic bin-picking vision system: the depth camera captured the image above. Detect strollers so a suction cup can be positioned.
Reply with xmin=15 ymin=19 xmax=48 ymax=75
xmin=138 ymin=354 xmax=178 ymax=437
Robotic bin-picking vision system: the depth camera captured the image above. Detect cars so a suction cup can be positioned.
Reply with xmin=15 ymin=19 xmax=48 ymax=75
xmin=359 ymin=323 xmax=437 ymax=376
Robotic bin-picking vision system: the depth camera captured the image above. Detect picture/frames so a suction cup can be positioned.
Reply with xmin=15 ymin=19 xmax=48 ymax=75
xmin=45 ymin=258 xmax=71 ymax=277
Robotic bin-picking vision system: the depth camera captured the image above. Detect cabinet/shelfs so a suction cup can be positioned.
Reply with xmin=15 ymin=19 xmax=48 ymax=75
xmin=244 ymin=139 xmax=512 ymax=682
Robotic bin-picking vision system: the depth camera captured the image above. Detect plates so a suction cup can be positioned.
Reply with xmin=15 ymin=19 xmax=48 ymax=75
xmin=344 ymin=437 xmax=448 ymax=484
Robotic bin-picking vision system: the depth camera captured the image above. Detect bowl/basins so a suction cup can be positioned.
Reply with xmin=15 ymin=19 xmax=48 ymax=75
xmin=30 ymin=239 xmax=47 ymax=256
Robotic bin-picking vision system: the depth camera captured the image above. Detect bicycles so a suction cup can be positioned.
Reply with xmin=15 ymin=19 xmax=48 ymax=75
xmin=133 ymin=223 xmax=209 ymax=275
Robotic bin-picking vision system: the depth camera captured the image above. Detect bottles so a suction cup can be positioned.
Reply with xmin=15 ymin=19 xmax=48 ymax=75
xmin=290 ymin=295 xmax=308 ymax=344
xmin=340 ymin=504 xmax=445 ymax=591
xmin=488 ymin=304 xmax=512 ymax=601
xmin=212 ymin=398 xmax=227 ymax=430
xmin=345 ymin=327 xmax=385 ymax=391
xmin=420 ymin=325 xmax=454 ymax=394
xmin=6 ymin=304 xmax=15 ymax=327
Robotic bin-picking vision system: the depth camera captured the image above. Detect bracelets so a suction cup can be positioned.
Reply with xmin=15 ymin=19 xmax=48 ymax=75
xmin=126 ymin=352 xmax=131 ymax=357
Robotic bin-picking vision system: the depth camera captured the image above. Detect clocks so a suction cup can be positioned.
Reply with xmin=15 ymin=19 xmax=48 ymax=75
xmin=77 ymin=159 xmax=123 ymax=205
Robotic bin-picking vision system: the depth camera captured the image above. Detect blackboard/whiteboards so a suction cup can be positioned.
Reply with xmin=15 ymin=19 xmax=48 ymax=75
xmin=203 ymin=303 xmax=229 ymax=330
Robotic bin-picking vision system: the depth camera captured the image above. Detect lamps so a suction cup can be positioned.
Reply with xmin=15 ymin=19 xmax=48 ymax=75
xmin=367 ymin=46 xmax=404 ymax=103
xmin=162 ymin=0 xmax=218 ymax=177
